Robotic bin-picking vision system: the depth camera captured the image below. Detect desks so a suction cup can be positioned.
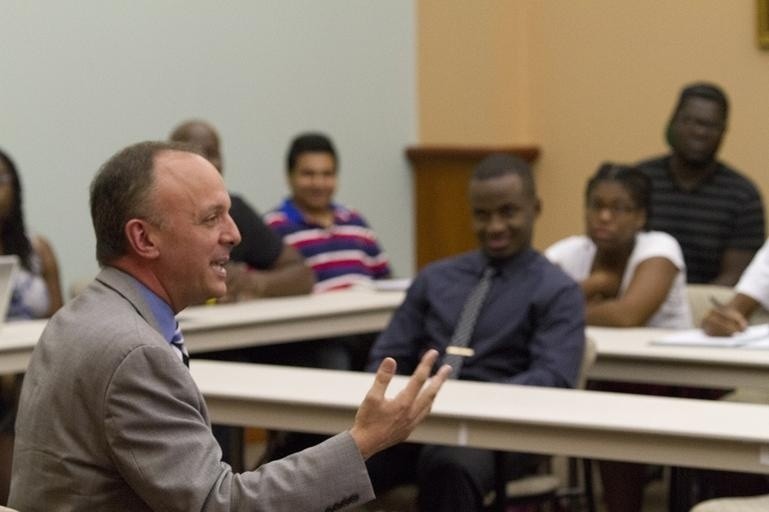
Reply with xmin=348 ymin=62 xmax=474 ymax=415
xmin=0 ymin=284 xmax=769 ymax=509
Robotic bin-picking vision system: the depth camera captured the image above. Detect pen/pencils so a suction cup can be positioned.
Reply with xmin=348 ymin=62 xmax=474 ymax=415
xmin=707 ymin=294 xmax=723 ymax=308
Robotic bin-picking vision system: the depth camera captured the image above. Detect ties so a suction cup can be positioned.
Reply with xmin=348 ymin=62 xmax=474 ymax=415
xmin=171 ymin=321 xmax=189 ymax=367
xmin=438 ymin=267 xmax=498 ymax=379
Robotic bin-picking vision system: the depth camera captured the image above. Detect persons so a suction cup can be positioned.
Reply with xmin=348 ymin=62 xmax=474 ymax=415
xmin=635 ymin=79 xmax=769 ymax=287
xmin=253 ymin=148 xmax=590 ymax=512
xmin=167 ymin=119 xmax=314 ymax=301
xmin=257 ymin=128 xmax=395 ymax=373
xmin=0 ymin=150 xmax=67 ymax=321
xmin=698 ymin=238 xmax=769 ymax=339
xmin=534 ymin=160 xmax=693 ymax=512
xmin=3 ymin=139 xmax=453 ymax=511
